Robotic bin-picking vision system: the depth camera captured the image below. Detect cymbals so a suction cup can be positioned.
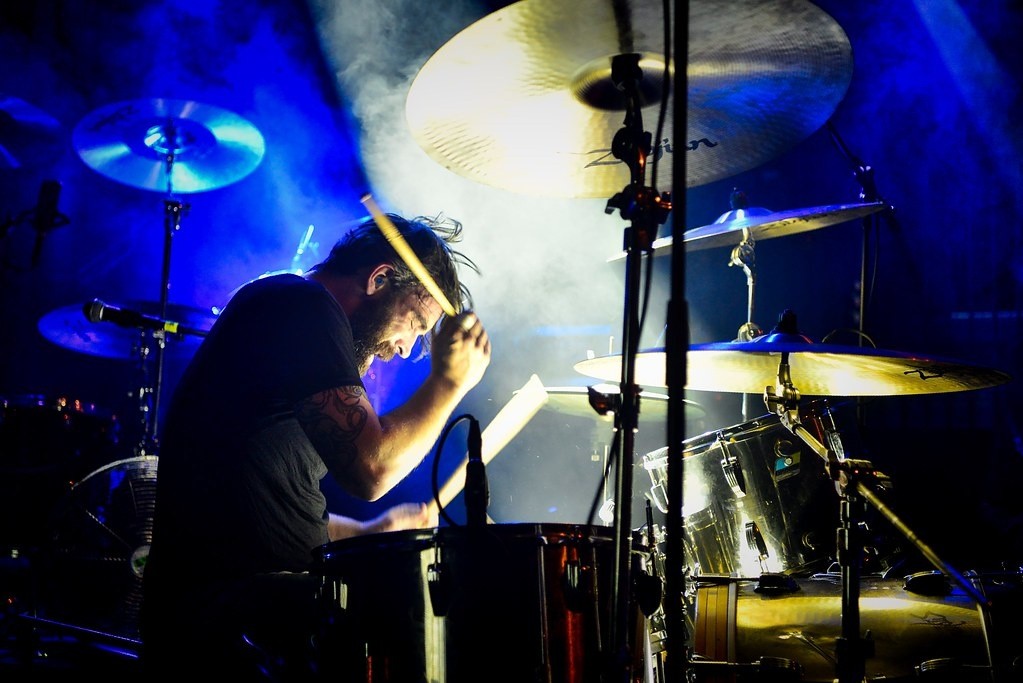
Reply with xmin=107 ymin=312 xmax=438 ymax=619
xmin=606 ymin=199 xmax=888 ymax=262
xmin=70 ymin=97 xmax=266 ymax=192
xmin=35 ymin=297 xmax=218 ymax=357
xmin=404 ymin=0 xmax=852 ymax=197
xmin=573 ymin=333 xmax=1013 ymax=395
xmin=519 ymin=383 xmax=707 ymax=424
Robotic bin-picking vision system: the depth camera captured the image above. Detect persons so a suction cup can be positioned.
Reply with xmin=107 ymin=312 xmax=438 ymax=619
xmin=140 ymin=211 xmax=492 ymax=683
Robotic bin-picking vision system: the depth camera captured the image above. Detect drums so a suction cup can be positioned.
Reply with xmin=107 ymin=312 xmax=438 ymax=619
xmin=643 ymin=401 xmax=848 ymax=585
xmin=1 ymin=378 xmax=136 ymax=566
xmin=328 ymin=522 xmax=643 ymax=683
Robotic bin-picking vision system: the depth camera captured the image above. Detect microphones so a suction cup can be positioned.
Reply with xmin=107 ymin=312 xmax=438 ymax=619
xmin=31 ymin=179 xmax=62 ymax=264
xmin=83 ymin=297 xmax=186 ymax=335
